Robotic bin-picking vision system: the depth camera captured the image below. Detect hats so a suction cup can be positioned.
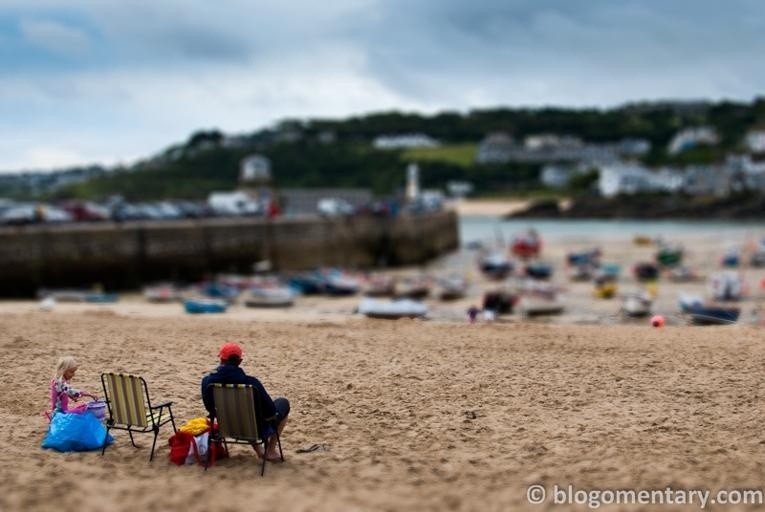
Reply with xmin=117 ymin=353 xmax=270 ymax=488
xmin=217 ymin=342 xmax=242 ymax=360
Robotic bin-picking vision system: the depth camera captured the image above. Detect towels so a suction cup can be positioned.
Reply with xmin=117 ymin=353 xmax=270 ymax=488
xmin=178 ymin=414 xmax=209 ymax=435
xmin=197 ymin=431 xmax=209 ymax=456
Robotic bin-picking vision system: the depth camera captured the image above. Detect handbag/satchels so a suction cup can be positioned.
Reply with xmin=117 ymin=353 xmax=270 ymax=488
xmin=167 ymin=419 xmax=230 ymax=466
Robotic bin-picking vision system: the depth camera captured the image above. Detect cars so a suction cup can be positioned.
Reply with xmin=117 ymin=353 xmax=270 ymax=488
xmin=2 ymin=187 xmax=450 ymax=228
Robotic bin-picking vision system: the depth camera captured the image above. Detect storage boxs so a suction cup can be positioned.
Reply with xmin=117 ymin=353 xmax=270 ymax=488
xmin=87 ymin=403 xmax=105 ymax=418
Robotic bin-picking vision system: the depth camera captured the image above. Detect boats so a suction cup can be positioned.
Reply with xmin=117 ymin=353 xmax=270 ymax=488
xmin=44 ymin=223 xmax=763 ymax=326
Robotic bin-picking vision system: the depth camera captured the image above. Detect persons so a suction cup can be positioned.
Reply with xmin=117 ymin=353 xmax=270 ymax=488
xmin=48 ymin=355 xmax=99 ymax=415
xmin=198 ymin=340 xmax=290 ymax=464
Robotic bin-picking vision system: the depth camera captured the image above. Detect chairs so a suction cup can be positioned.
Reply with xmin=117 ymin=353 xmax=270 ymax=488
xmin=200 ymin=382 xmax=288 ymax=476
xmin=97 ymin=372 xmax=180 ymax=462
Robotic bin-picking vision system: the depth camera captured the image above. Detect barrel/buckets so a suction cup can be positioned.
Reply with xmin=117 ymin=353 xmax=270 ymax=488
xmin=85 ymin=400 xmax=106 ymax=421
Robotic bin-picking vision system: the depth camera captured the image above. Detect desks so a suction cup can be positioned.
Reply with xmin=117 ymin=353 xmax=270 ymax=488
xmin=173 ymin=418 xmax=229 ymax=469
